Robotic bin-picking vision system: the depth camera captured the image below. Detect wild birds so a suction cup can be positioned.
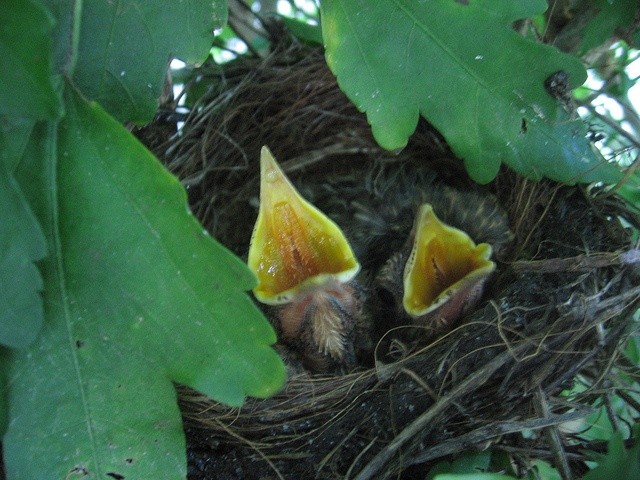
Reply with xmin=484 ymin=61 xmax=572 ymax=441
xmin=244 ymin=146 xmax=370 ymax=377
xmin=352 ymin=188 xmax=516 ymax=348
